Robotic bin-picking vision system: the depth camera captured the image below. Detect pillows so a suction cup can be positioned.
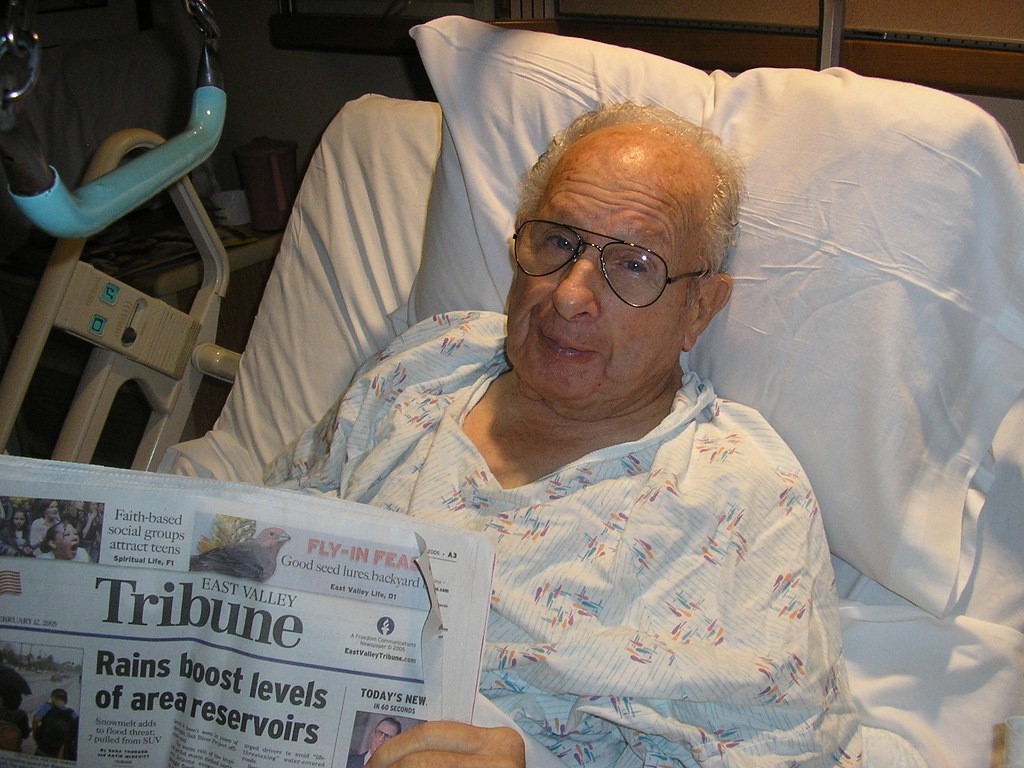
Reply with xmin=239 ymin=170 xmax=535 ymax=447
xmin=384 ymin=14 xmax=1023 ymax=622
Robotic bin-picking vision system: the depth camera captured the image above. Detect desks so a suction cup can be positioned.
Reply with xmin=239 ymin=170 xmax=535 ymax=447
xmin=0 ymin=222 xmax=284 ymax=457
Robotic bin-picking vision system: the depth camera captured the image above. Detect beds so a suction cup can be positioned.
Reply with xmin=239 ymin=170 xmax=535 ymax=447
xmin=0 ymin=90 xmax=1024 ymax=767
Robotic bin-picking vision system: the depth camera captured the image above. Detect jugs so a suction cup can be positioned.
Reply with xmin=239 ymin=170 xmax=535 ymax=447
xmin=234 ymin=136 xmax=298 ymax=231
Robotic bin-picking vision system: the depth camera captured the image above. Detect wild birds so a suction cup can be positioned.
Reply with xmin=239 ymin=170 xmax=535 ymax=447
xmin=189 ymin=524 xmax=293 ymax=583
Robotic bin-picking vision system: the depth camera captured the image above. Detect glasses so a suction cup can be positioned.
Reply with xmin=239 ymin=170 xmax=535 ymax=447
xmin=513 ymin=220 xmax=711 ymax=308
xmin=374 ymin=728 xmax=392 ymax=741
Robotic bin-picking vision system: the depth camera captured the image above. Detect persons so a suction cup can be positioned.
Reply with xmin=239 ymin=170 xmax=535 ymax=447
xmin=0 ymin=642 xmax=79 ymax=762
xmin=260 ymin=101 xmax=862 ymax=768
xmin=345 ymin=716 xmax=402 ymax=768
xmin=0 ymin=496 xmax=104 ymax=565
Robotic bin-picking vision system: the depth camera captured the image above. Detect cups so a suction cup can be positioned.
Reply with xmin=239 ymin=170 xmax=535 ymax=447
xmin=209 ymin=190 xmax=251 ymax=226
